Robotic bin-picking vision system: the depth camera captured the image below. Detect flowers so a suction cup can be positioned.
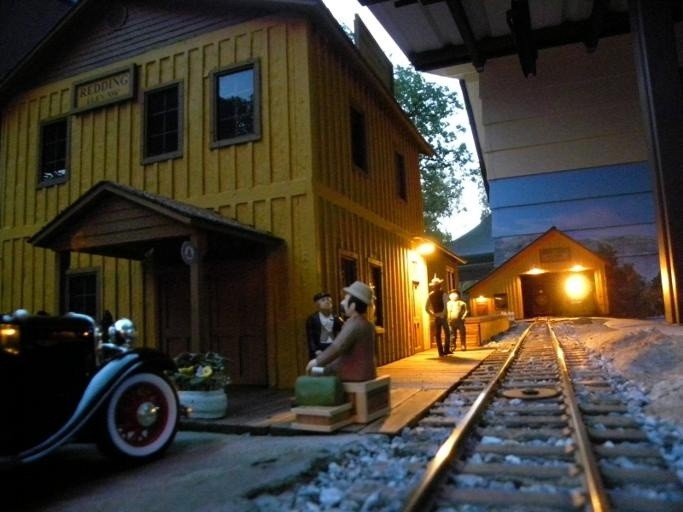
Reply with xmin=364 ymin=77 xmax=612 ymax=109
xmin=157 ymin=348 xmax=236 ymax=392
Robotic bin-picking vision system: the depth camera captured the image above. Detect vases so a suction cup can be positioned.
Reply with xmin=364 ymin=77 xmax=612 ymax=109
xmin=172 ymin=385 xmax=229 ymax=422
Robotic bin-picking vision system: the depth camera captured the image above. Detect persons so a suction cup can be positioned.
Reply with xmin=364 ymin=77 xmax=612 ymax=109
xmin=424 ymin=276 xmax=455 ymax=357
xmin=305 ymin=290 xmax=343 ymax=359
xmin=305 ymin=280 xmax=380 ymax=382
xmin=444 ymin=287 xmax=469 ymax=353
